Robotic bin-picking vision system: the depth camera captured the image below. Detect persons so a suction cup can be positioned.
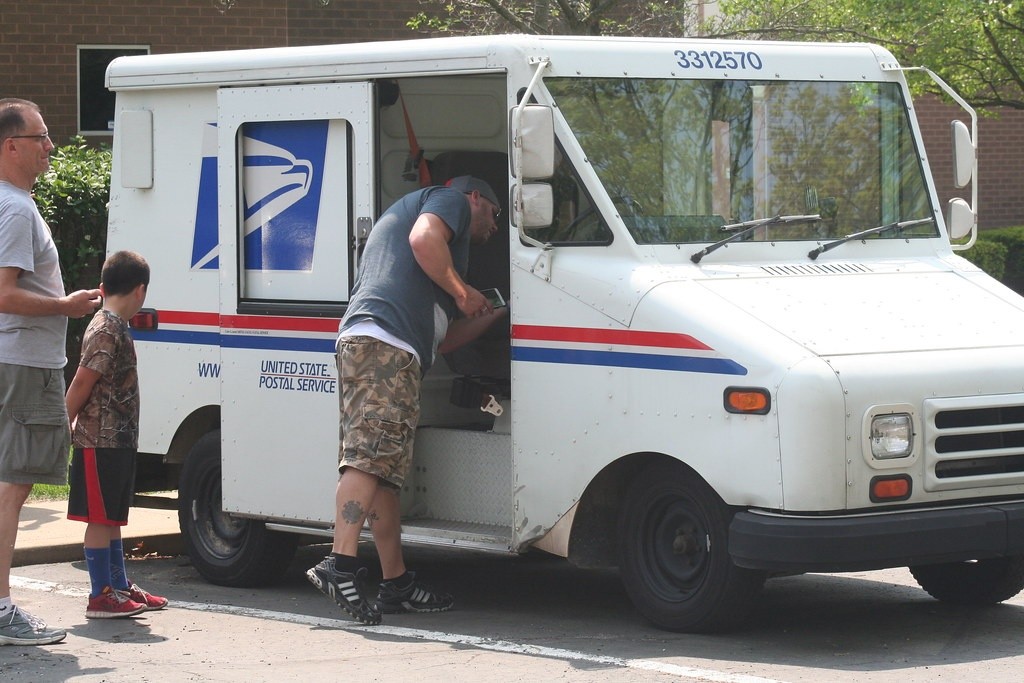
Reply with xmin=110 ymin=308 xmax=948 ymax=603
xmin=66 ymin=251 xmax=169 ymax=619
xmin=0 ymin=98 xmax=102 ymax=646
xmin=305 ymin=174 xmax=510 ymax=625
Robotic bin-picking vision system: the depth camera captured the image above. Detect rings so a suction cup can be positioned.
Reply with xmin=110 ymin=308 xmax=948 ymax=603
xmin=480 ymin=307 xmax=488 ymax=311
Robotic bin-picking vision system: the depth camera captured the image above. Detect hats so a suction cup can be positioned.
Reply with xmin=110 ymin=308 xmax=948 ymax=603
xmin=446 ymin=176 xmax=499 ymax=208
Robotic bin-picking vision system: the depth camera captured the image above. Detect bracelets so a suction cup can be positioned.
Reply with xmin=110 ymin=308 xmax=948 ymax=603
xmin=505 ymin=304 xmax=510 ymax=311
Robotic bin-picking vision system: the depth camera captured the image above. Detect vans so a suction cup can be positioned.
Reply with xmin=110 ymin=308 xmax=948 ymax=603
xmin=104 ymin=34 xmax=1024 ymax=637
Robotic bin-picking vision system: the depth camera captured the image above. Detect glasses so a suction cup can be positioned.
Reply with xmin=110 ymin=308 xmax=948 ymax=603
xmin=2 ymin=133 xmax=48 ymax=149
xmin=479 ymin=198 xmax=501 ymax=217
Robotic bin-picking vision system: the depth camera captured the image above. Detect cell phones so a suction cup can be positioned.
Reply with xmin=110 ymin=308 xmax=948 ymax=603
xmin=479 ymin=288 xmax=506 ymax=309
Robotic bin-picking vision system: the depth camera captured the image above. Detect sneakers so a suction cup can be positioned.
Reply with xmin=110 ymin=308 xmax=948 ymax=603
xmin=0 ymin=605 xmax=66 ymax=646
xmin=86 ymin=587 xmax=148 ymax=618
xmin=305 ymin=557 xmax=382 ymax=625
xmin=126 ymin=580 xmax=168 ymax=610
xmin=373 ymin=572 xmax=454 ymax=614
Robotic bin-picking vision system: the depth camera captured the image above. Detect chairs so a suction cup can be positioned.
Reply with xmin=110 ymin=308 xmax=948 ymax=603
xmin=427 ymin=147 xmax=509 ymax=391
